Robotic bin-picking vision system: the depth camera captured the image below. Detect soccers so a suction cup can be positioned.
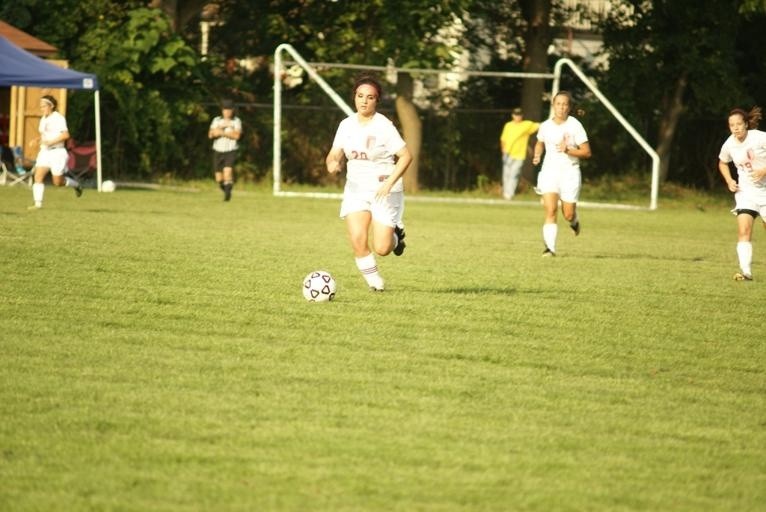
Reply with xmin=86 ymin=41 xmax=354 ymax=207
xmin=102 ymin=180 xmax=116 ymax=193
xmin=303 ymin=271 xmax=336 ymax=301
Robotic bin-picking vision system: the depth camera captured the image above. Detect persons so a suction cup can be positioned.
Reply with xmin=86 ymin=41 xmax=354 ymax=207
xmin=29 ymin=94 xmax=83 ymax=213
xmin=207 ymin=99 xmax=244 ymax=203
xmin=718 ymin=107 xmax=766 ymax=282
xmin=499 ymin=90 xmax=592 ymax=258
xmin=324 ymin=76 xmax=414 ymax=292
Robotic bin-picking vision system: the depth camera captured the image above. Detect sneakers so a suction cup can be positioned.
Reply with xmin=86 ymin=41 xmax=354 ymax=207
xmin=393 ymin=225 xmax=405 ymax=256
xmin=569 ymin=220 xmax=580 ymax=237
xmin=541 ymin=249 xmax=556 ymax=257
xmin=74 ymin=183 xmax=82 ymax=197
xmin=732 ymin=273 xmax=753 ymax=282
xmin=369 ymin=286 xmax=384 ymax=293
